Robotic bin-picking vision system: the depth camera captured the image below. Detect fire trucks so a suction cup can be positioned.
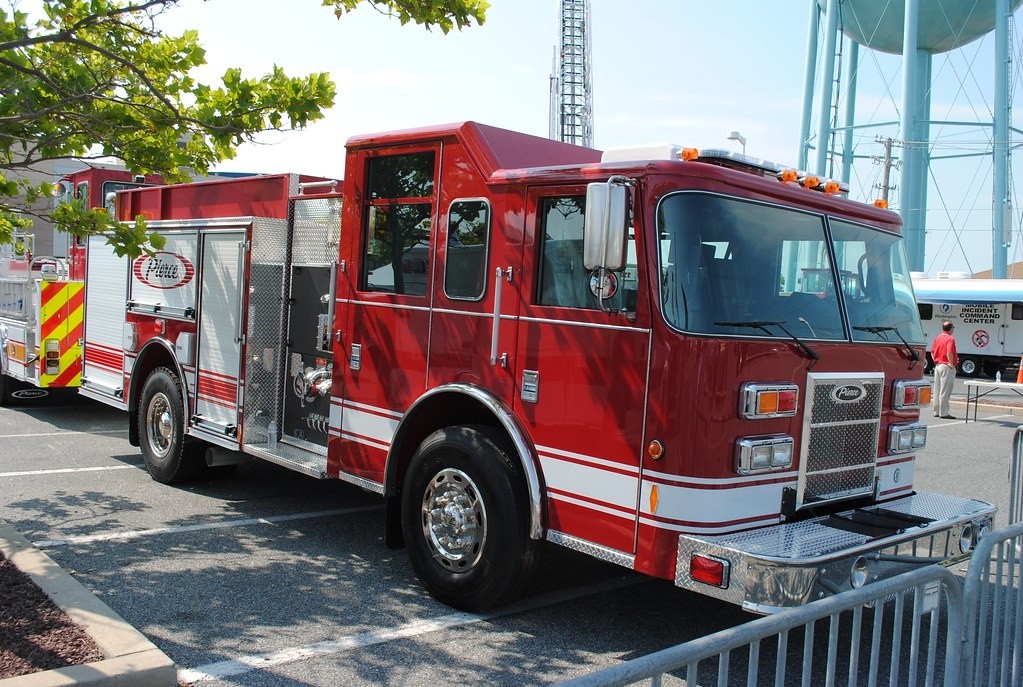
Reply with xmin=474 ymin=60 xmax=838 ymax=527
xmin=47 ymin=117 xmax=999 ymax=628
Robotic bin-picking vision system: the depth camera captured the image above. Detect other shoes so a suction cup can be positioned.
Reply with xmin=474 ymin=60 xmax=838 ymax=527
xmin=940 ymin=415 xmax=957 ymax=419
xmin=934 ymin=415 xmax=940 ymax=417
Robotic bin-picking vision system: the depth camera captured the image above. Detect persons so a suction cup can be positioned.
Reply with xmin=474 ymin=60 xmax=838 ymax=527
xmin=931 ymin=321 xmax=958 ymax=419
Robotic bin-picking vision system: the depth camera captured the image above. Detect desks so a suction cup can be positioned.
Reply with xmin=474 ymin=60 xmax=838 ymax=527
xmin=964 ymin=381 xmax=1023 ymax=424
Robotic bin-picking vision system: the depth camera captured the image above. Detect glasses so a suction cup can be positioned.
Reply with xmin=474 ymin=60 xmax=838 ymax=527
xmin=951 ymin=326 xmax=955 ymax=329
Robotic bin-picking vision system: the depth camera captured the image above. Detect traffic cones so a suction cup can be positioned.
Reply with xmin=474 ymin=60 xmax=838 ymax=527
xmin=1016 ymin=356 xmax=1023 ymax=384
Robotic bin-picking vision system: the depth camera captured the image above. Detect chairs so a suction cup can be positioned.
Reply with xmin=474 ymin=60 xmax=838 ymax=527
xmin=711 ymin=257 xmax=766 ymax=338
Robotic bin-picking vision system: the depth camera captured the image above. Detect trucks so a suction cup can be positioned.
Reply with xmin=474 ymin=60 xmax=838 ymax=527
xmin=0 ymin=207 xmax=82 ymax=403
